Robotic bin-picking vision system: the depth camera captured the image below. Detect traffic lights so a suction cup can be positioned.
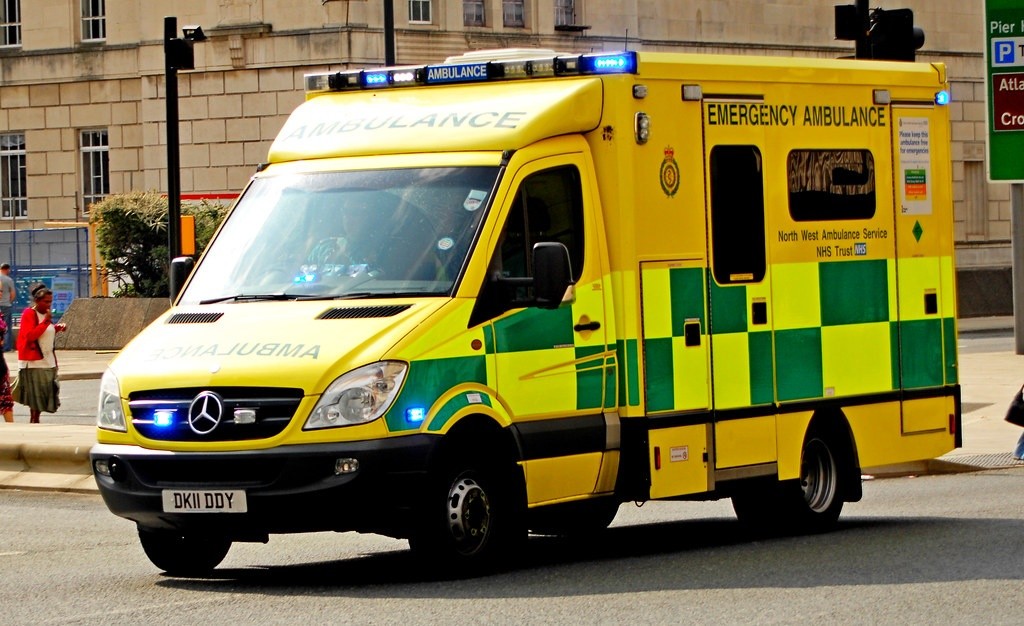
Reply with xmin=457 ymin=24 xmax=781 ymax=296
xmin=854 ymin=4 xmax=926 ymax=63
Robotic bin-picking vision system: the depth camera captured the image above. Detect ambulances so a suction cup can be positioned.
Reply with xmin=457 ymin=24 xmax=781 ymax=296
xmin=83 ymin=49 xmax=960 ymax=577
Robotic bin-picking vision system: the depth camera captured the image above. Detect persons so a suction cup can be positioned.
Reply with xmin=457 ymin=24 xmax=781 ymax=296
xmin=10 ymin=284 xmax=66 ymax=423
xmin=306 ymin=191 xmax=394 ymax=281
xmin=0 ymin=263 xmax=16 ymax=352
xmin=0 ymin=279 xmax=14 ymax=422
